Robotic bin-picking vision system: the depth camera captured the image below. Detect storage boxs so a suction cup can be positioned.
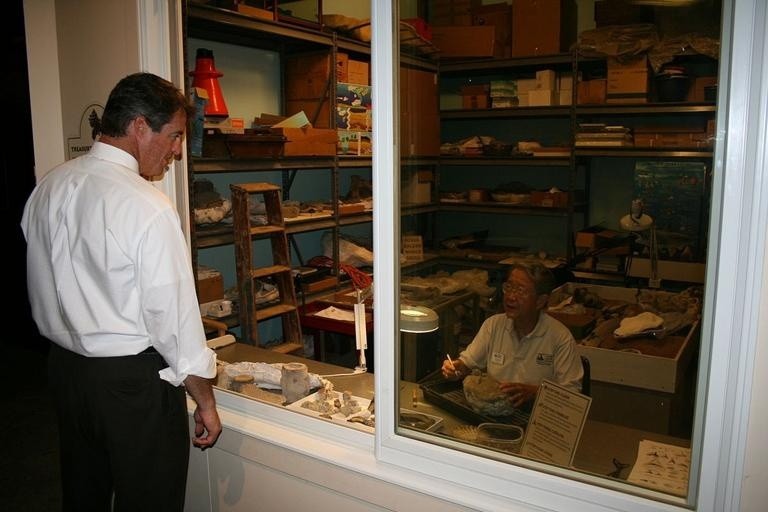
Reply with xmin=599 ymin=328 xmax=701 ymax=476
xmin=545 ymin=282 xmax=697 ymax=434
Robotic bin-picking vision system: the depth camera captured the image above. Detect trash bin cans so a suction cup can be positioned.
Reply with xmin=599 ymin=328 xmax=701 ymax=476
xmin=400 ymin=304 xmax=439 ymax=382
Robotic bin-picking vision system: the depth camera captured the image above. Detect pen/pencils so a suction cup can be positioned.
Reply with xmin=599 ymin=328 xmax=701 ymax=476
xmin=447 ymin=354 xmax=459 ymax=377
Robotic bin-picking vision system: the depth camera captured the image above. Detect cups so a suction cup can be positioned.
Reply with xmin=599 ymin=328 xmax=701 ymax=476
xmin=703 ymin=85 xmax=717 ymax=102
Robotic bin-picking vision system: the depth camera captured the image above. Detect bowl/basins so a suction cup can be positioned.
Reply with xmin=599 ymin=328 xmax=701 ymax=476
xmin=654 ymin=74 xmax=687 ymax=102
xmin=438 ymin=143 xmax=515 ymax=159
xmin=437 ymin=187 xmax=528 ymax=203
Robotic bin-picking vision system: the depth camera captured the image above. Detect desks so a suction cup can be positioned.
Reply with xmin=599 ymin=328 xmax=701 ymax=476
xmin=209 ymin=336 xmax=690 ymax=502
xmin=302 ymin=271 xmax=477 ymax=382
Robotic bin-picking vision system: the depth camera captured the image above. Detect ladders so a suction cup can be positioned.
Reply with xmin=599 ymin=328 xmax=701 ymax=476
xmin=230 ymin=183 xmax=305 ymax=358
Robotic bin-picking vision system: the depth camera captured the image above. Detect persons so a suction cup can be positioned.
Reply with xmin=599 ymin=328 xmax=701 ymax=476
xmin=440 ymin=256 xmax=586 ymax=408
xmin=18 ymin=68 xmax=224 ymax=511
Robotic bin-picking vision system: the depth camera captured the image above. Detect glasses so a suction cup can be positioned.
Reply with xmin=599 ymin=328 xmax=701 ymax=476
xmin=501 ymin=280 xmax=538 ymax=298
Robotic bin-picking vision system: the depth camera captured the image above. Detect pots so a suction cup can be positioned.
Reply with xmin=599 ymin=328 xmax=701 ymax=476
xmin=202 ymin=127 xmax=287 ymax=160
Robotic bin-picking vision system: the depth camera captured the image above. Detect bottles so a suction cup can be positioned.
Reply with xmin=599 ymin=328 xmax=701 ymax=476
xmin=185 ymin=48 xmax=229 ymax=119
xmin=232 ymin=374 xmax=256 ymax=396
xmin=280 ymin=362 xmax=311 ymax=404
